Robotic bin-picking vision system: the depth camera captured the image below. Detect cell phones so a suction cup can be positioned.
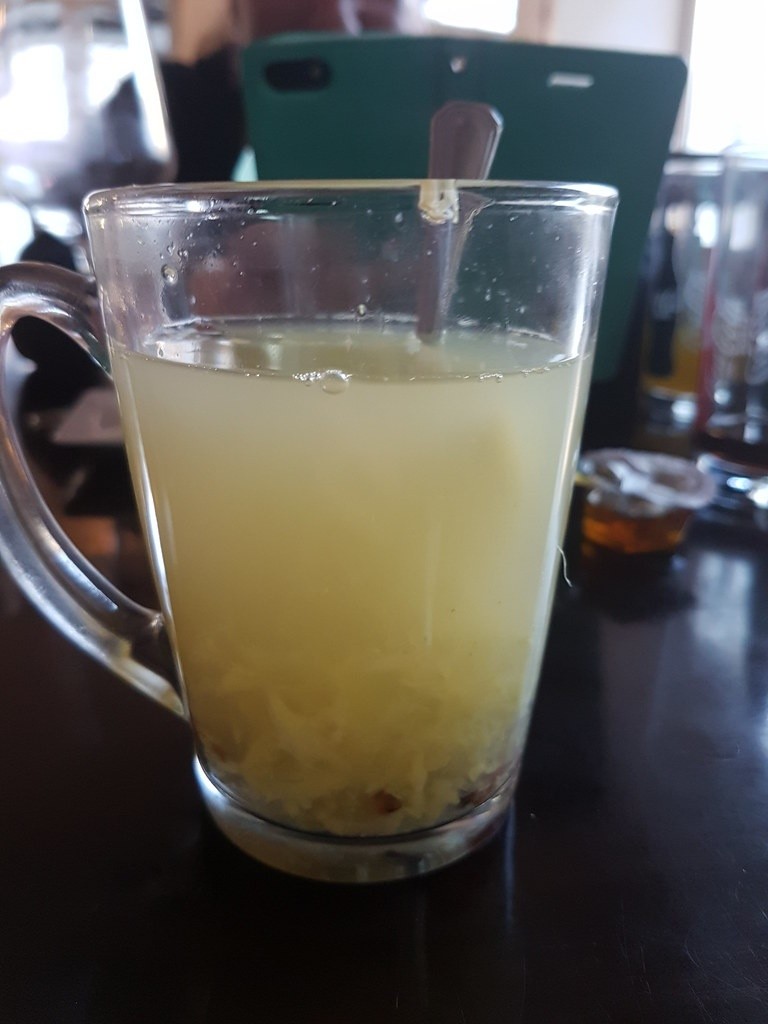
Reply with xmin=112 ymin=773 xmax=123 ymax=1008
xmin=242 ymin=32 xmax=688 ymax=379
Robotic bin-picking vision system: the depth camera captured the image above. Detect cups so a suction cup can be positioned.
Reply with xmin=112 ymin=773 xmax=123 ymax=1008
xmin=0 ymin=177 xmax=620 ymax=886
